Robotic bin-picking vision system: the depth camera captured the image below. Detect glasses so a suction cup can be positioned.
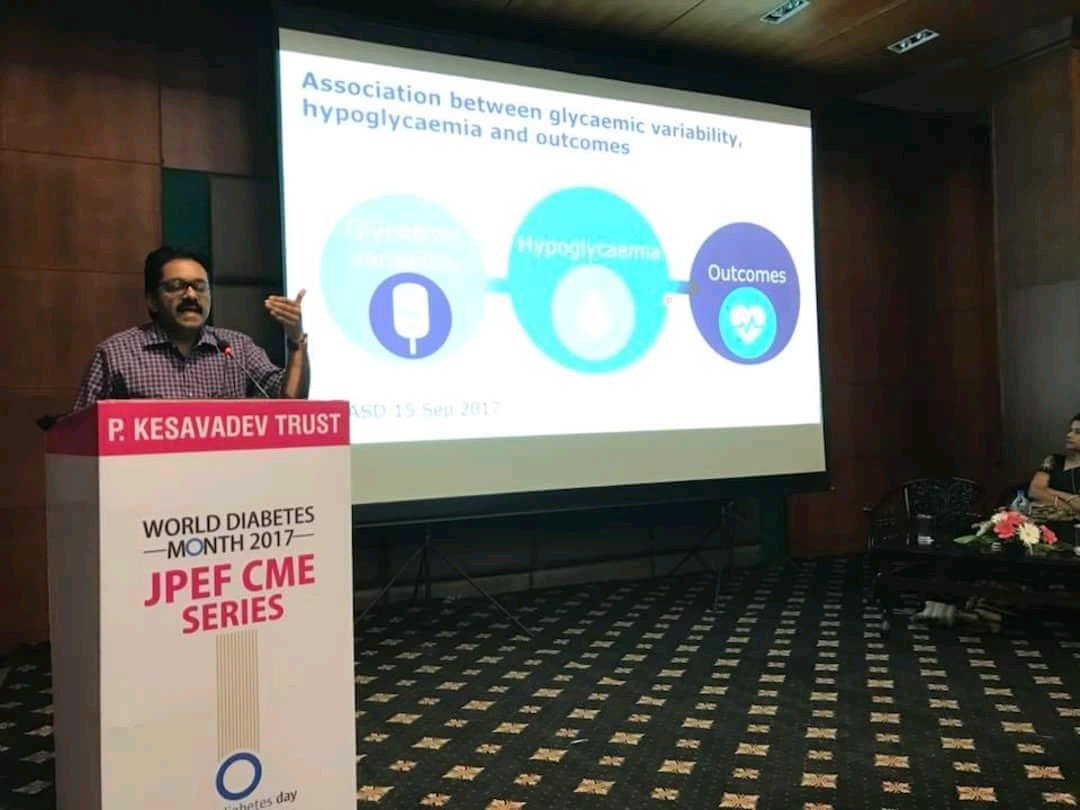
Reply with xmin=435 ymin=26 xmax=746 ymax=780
xmin=158 ymin=279 xmax=209 ymax=292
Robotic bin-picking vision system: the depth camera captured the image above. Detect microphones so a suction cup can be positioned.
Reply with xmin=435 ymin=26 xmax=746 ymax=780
xmin=217 ymin=339 xmax=269 ymax=398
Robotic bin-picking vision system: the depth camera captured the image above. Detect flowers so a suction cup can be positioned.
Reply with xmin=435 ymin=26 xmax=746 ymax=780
xmin=954 ymin=511 xmax=1062 ymax=555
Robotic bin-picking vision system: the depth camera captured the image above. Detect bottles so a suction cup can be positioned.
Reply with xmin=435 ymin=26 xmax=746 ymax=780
xmin=1012 ymin=490 xmax=1030 ymax=512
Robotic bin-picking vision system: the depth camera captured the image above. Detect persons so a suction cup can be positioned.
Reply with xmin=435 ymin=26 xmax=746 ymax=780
xmin=1028 ymin=414 xmax=1080 ymax=513
xmin=72 ymin=245 xmax=306 ymax=419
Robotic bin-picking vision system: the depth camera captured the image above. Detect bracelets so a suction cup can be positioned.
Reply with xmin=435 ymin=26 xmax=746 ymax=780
xmin=287 ymin=333 xmax=308 ymax=350
xmin=1054 ymin=496 xmax=1057 ymax=506
xmin=1065 ymin=495 xmax=1079 ymax=503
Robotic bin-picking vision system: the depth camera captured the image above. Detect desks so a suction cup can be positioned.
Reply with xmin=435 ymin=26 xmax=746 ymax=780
xmin=871 ymin=545 xmax=1079 ymax=609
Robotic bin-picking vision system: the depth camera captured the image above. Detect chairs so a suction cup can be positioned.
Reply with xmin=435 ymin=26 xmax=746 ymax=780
xmin=863 ymin=476 xmax=982 ymax=572
